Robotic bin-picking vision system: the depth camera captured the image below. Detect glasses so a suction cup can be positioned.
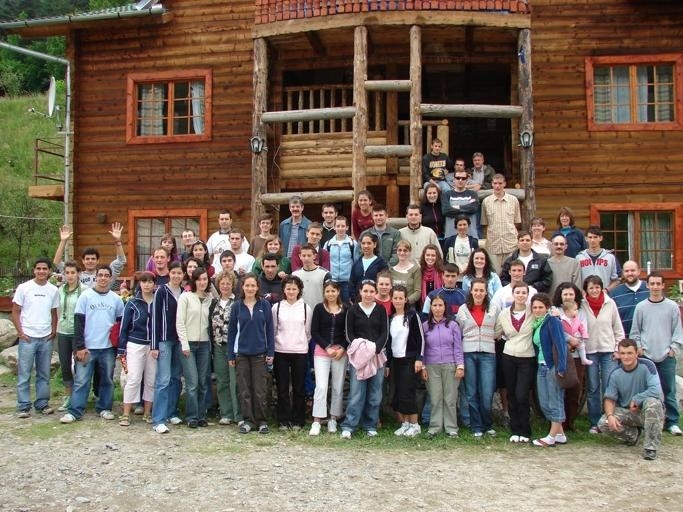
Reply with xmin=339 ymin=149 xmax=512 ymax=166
xmin=324 ymin=279 xmax=338 ymax=283
xmin=362 ymin=279 xmax=375 ymax=284
xmin=455 ymin=177 xmax=467 ymax=180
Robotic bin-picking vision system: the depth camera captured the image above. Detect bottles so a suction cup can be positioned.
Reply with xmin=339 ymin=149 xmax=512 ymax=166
xmin=266 ymin=358 xmax=272 ymax=372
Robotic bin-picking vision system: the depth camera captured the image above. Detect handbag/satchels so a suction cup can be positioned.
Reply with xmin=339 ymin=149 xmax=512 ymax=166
xmin=552 ymin=342 xmax=580 ymax=390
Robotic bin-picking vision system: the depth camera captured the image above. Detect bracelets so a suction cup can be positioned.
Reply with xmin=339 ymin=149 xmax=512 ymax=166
xmin=112 ymin=239 xmax=122 ymax=249
xmin=18 ymin=333 xmax=25 ymax=338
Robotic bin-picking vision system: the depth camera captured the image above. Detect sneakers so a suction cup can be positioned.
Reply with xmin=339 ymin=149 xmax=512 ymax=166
xmin=219 ymin=417 xmax=302 ymax=434
xmin=668 ymin=425 xmax=683 ymax=436
xmin=308 ymin=419 xmax=378 ymax=440
xmin=16 ymin=392 xmax=115 ymax=423
xmin=627 ymin=427 xmax=657 ymax=460
xmin=118 ymin=406 xmax=208 ymax=433
xmin=589 ymin=426 xmax=600 ymax=433
xmin=394 ymin=421 xmax=498 ymax=439
xmin=509 ymin=434 xmax=567 ymax=447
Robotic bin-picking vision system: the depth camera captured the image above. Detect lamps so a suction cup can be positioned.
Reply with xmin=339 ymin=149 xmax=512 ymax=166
xmin=515 ymin=128 xmax=535 ymax=150
xmin=246 ymin=131 xmax=269 ymax=157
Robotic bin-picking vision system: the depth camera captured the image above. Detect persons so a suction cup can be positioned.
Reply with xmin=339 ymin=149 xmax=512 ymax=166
xmin=465 ymin=152 xmax=496 ymax=190
xmin=54 ymin=260 xmax=92 ymax=411
xmin=479 ymin=175 xmax=523 ymax=265
xmin=52 ymin=221 xmax=128 ymax=401
xmin=10 ymin=260 xmax=58 ymax=420
xmin=419 ymin=185 xmax=445 ymax=238
xmin=422 ymin=139 xmax=453 ymax=188
xmin=441 ymin=170 xmax=483 ymax=240
xmin=440 ymin=158 xmax=481 ymax=192
xmin=118 ymin=191 xmax=681 ymax=460
xmin=60 ymin=265 xmax=124 ymax=424
